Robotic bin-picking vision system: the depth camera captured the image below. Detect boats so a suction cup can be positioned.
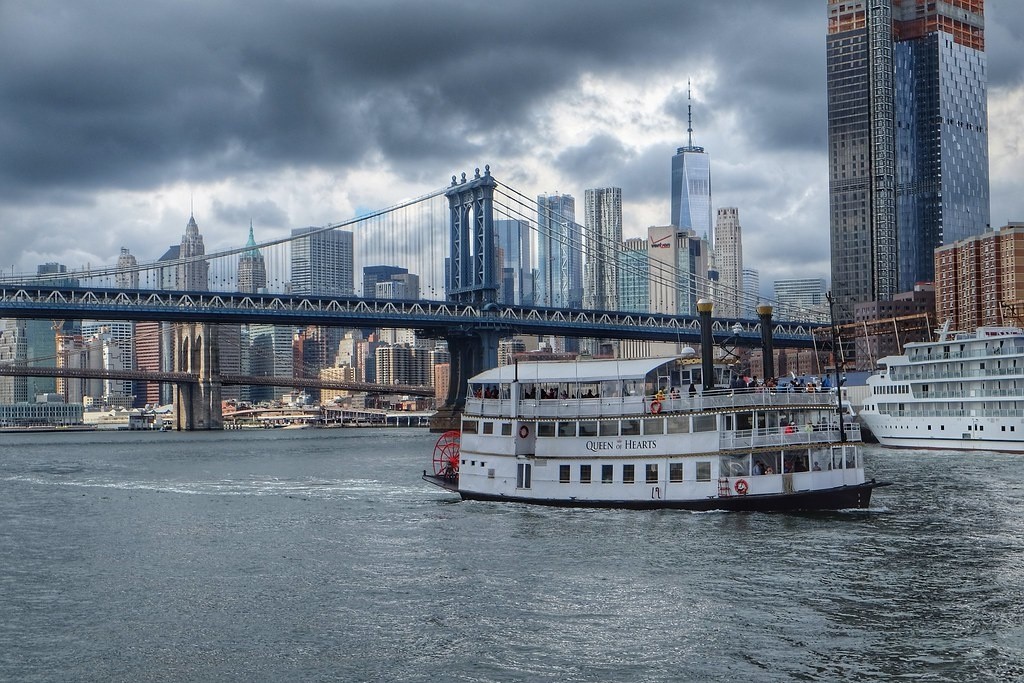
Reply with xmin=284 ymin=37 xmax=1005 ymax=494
xmin=118 ymin=411 xmax=171 ymax=430
xmin=859 ymin=305 xmax=1024 ymax=450
xmin=420 ymin=298 xmax=892 ymax=513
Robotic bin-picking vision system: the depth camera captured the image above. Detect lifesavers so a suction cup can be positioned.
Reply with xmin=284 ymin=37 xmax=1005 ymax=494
xmin=519 ymin=425 xmax=528 ymax=438
xmin=651 ymin=401 xmax=661 ymax=413
xmin=734 ymin=480 xmax=748 ymax=493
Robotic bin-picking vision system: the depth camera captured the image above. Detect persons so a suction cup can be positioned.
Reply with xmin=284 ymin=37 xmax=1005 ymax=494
xmin=475 ymin=382 xmax=697 ymax=415
xmin=729 ymin=373 xmax=859 ymax=476
xmin=991 ymin=346 xmax=1000 ymax=354
